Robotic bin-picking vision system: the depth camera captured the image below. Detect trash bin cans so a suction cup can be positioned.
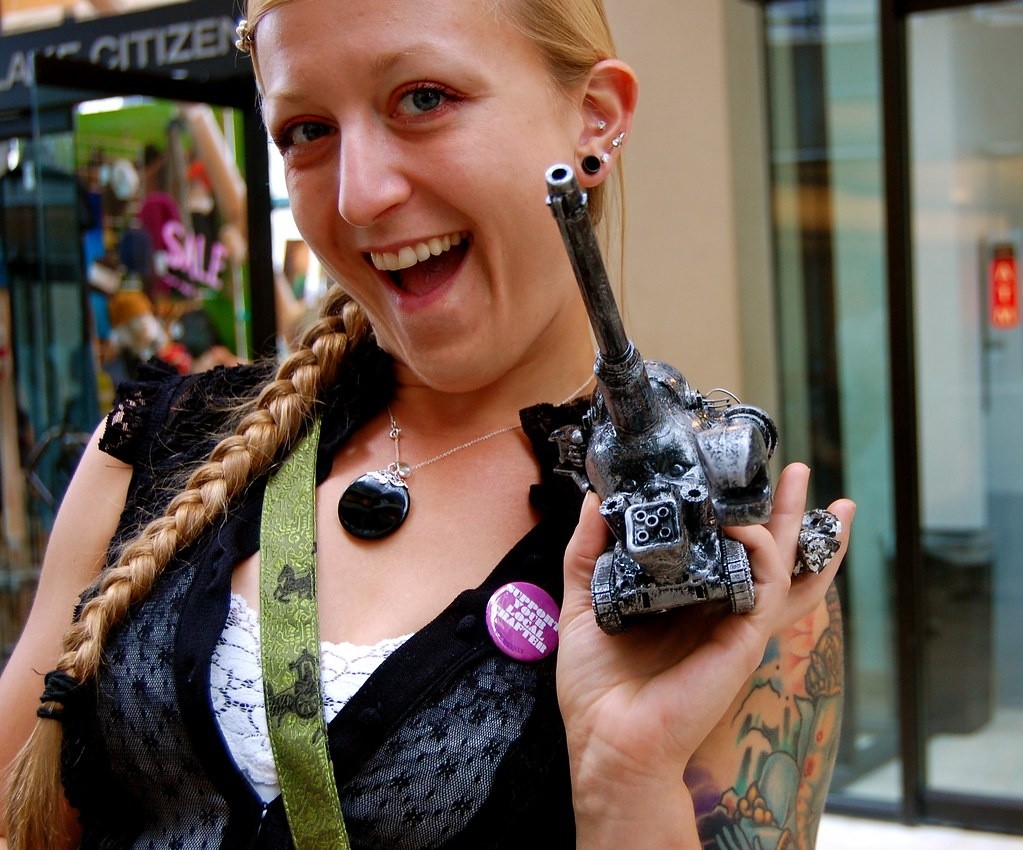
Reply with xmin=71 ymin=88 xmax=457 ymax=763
xmin=921 ymin=529 xmax=993 ymax=733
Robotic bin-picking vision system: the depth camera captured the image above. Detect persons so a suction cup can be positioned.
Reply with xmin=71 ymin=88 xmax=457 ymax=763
xmin=0 ymin=0 xmax=859 ymax=850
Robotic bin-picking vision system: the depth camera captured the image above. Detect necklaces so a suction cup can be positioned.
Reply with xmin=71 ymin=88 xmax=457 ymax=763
xmin=337 ymin=372 xmax=600 ymax=540
